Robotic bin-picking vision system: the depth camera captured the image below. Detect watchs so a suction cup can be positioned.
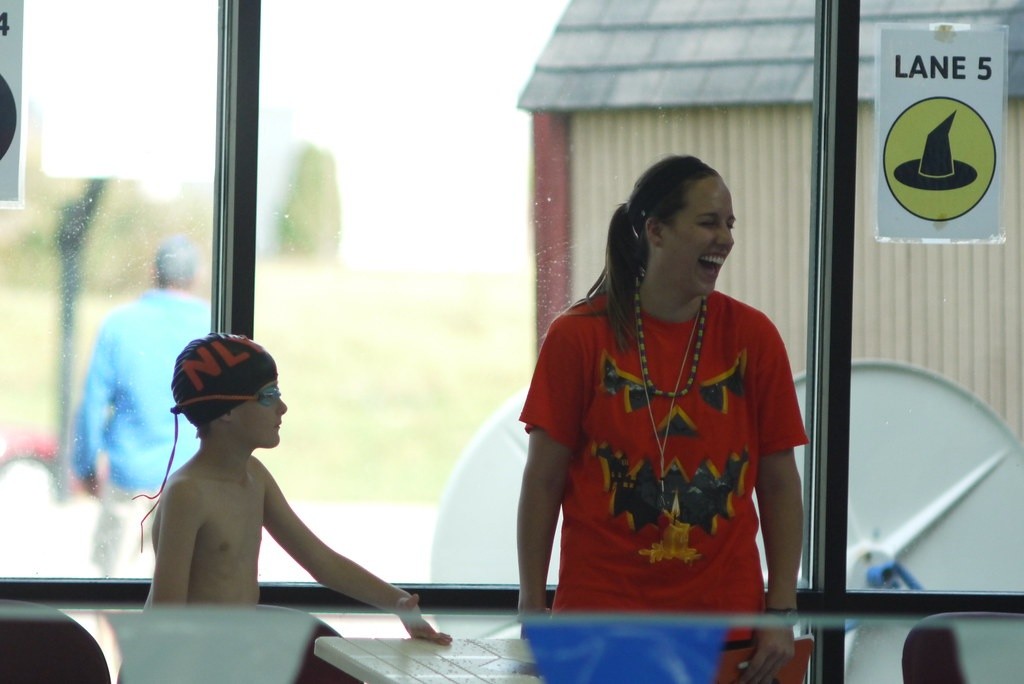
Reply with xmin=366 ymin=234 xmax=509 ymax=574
xmin=764 ymin=607 xmax=799 ymax=616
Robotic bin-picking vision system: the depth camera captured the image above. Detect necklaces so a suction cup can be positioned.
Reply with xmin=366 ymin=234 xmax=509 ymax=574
xmin=635 ymin=273 xmax=706 ymax=399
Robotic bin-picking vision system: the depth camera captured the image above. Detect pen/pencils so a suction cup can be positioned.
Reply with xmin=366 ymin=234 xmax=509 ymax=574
xmin=738 ymin=661 xmax=750 ymax=670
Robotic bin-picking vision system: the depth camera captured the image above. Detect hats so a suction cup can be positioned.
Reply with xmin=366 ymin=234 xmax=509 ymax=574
xmin=157 ymin=237 xmax=199 ymax=276
xmin=171 ymin=333 xmax=278 ymax=426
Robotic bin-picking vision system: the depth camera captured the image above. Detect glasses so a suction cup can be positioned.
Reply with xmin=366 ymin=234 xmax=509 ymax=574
xmin=176 ymin=386 xmax=281 ymax=406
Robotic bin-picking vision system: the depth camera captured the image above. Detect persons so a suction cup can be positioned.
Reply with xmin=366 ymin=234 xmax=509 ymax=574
xmin=517 ymin=157 xmax=810 ymax=683
xmin=73 ymin=236 xmax=212 ymax=577
xmin=142 ymin=334 xmax=453 ymax=646
